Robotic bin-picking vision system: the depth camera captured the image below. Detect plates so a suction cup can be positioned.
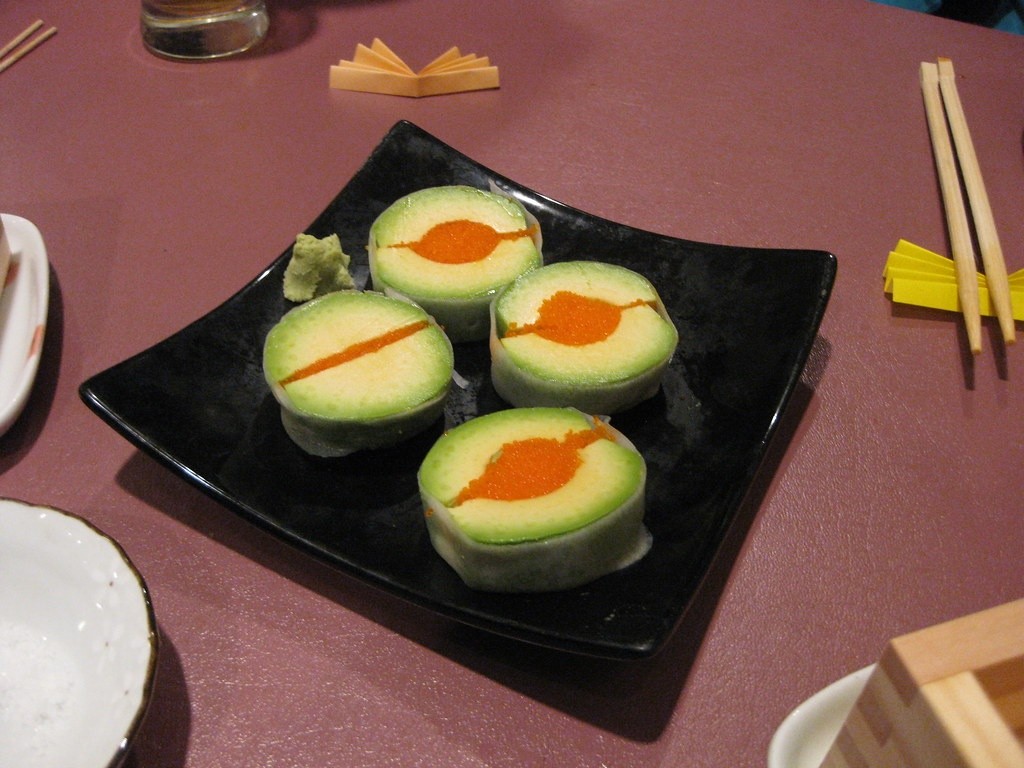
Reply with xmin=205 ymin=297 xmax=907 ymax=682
xmin=2 ymin=497 xmax=162 ymax=768
xmin=0 ymin=213 xmax=49 ymax=436
xmin=74 ymin=121 xmax=843 ymax=679
xmin=764 ymin=658 xmax=882 ymax=768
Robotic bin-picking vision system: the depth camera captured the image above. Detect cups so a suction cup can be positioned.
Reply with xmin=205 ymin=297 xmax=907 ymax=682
xmin=141 ymin=0 xmax=272 ymax=61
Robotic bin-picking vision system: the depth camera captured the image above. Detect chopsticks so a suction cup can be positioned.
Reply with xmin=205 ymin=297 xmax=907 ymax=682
xmin=918 ymin=54 xmax=1017 ymax=351
xmin=0 ymin=16 xmax=57 ymax=75
